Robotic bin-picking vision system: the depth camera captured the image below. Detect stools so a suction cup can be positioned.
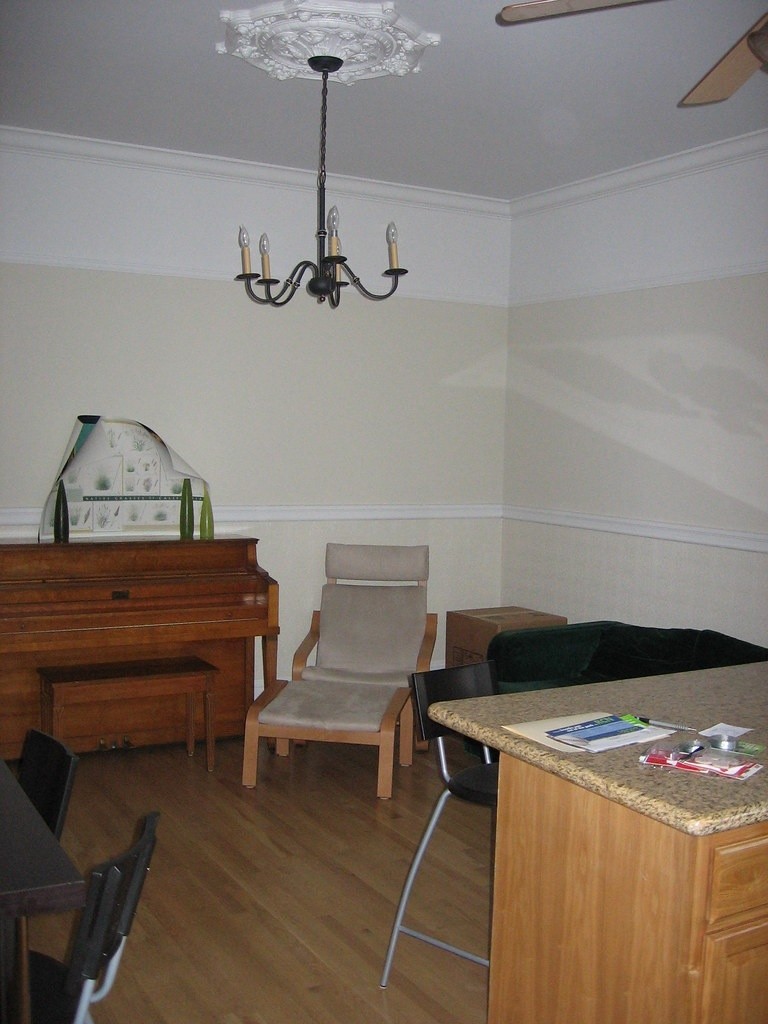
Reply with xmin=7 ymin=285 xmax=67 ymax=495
xmin=378 ymin=659 xmax=501 ymax=991
xmin=243 ymin=678 xmax=414 ymax=798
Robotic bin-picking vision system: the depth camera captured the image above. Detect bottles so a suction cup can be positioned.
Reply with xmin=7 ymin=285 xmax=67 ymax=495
xmin=180 ymin=479 xmax=194 ymax=537
xmin=200 ymin=480 xmax=215 ymax=540
xmin=53 ymin=479 xmax=70 ymax=544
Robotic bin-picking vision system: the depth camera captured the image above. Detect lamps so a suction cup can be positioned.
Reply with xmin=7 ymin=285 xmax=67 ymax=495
xmin=236 ymin=55 xmax=406 ymax=308
xmin=746 ymin=23 xmax=767 ymax=64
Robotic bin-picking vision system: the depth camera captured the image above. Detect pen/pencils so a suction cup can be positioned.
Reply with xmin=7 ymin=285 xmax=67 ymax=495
xmin=635 ymin=717 xmax=697 ymax=732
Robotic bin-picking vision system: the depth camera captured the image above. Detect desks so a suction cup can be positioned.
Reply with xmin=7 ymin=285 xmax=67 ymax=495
xmin=0 ymin=757 xmax=90 ymax=1024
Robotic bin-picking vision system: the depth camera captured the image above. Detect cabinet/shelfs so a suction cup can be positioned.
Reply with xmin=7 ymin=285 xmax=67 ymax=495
xmin=427 ymin=656 xmax=768 ymax=1024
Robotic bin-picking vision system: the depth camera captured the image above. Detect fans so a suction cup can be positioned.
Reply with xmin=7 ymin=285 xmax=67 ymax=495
xmin=501 ymin=0 xmax=767 ymax=107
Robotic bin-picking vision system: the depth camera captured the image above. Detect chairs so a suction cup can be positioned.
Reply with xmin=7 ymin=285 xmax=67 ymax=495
xmin=290 ymin=539 xmax=438 ymax=752
xmin=16 ymin=728 xmax=79 ymax=845
xmin=2 ymin=808 xmax=162 ymax=1024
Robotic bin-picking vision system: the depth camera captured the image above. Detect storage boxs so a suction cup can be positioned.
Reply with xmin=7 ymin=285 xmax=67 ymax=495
xmin=444 ymin=605 xmax=568 ymax=669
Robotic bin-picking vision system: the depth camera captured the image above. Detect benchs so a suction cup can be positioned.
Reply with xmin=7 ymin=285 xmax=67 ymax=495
xmin=37 ymin=655 xmax=220 ymax=772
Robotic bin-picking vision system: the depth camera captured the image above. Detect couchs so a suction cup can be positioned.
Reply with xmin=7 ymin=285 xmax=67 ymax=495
xmin=485 ymin=618 xmax=768 ymax=696
